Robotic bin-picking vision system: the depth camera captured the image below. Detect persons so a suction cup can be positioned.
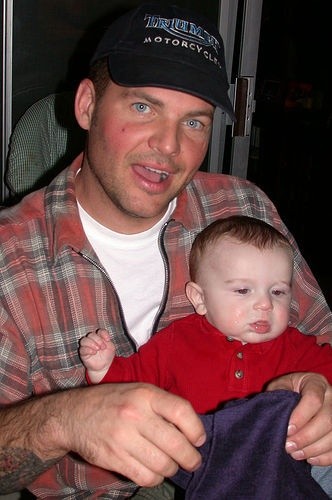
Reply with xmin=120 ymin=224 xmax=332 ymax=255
xmin=0 ymin=0 xmax=332 ymax=500
xmin=79 ymin=215 xmax=332 ymax=419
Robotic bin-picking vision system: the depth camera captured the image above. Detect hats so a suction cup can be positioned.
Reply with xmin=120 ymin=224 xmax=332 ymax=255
xmin=87 ymin=1 xmax=237 ymax=123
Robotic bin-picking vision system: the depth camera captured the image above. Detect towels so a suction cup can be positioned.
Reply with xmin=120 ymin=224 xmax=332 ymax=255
xmin=164 ymin=389 xmax=332 ymax=500
